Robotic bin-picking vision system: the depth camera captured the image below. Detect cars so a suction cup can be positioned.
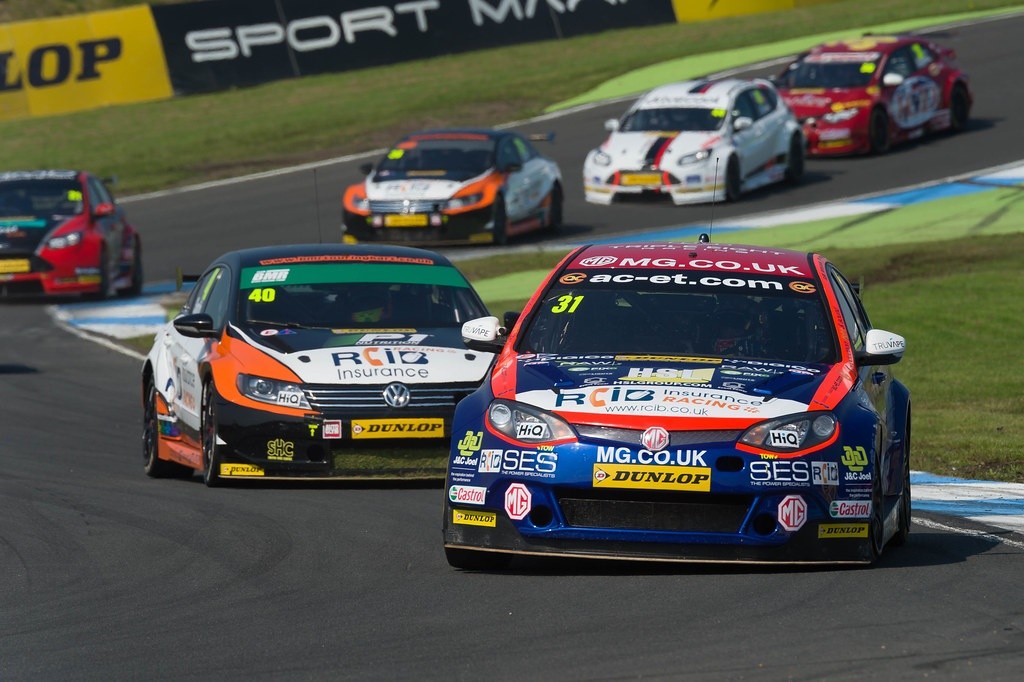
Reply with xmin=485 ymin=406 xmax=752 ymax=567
xmin=140 ymin=240 xmax=506 ymax=492
xmin=444 ymin=232 xmax=912 ymax=565
xmin=771 ymin=30 xmax=973 ymax=158
xmin=340 ymin=129 xmax=563 ymax=255
xmin=582 ymin=77 xmax=810 ymax=212
xmin=0 ymin=167 xmax=143 ymax=308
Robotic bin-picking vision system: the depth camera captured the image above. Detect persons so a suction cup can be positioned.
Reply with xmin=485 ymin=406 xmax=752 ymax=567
xmin=347 ymin=288 xmax=390 ymax=330
xmin=685 ymin=297 xmax=787 ymax=366
